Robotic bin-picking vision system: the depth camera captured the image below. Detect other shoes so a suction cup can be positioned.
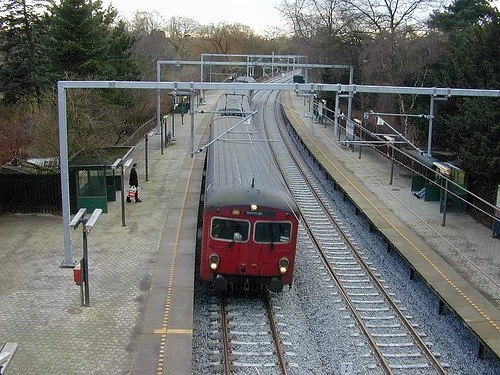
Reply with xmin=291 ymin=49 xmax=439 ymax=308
xmin=126 ymin=196 xmax=131 ymax=202
xmin=136 ymin=197 xmax=142 ymax=202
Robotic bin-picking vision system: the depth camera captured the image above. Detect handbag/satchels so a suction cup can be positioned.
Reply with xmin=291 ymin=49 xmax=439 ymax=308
xmin=128 ymin=185 xmax=137 ymax=197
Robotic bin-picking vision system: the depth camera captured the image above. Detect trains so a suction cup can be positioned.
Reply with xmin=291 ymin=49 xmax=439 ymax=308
xmin=198 ymin=76 xmax=299 ymax=296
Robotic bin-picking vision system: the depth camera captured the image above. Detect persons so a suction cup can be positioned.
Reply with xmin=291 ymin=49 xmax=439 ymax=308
xmin=318 ymin=98 xmax=324 ymax=124
xmin=126 ymin=163 xmax=142 ymax=203
xmin=413 ymin=187 xmax=425 ymax=198
